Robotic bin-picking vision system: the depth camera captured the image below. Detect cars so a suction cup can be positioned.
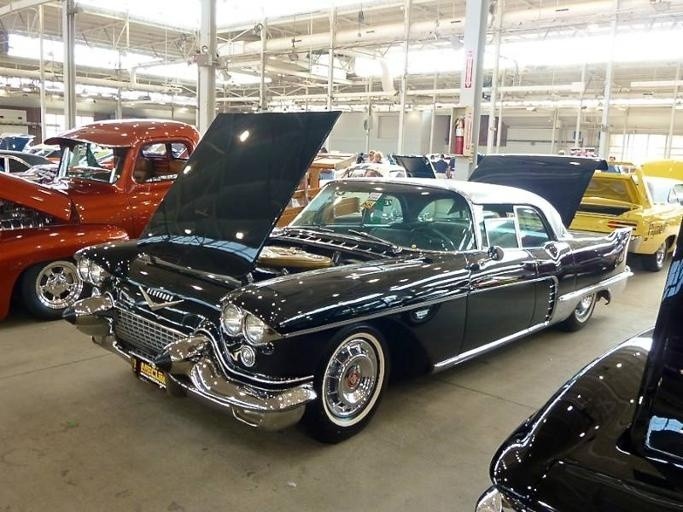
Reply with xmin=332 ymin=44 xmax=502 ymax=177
xmin=65 ymin=112 xmax=632 ymax=446
xmin=0 ymin=134 xmax=62 ymax=185
xmin=1 ymin=117 xmax=202 ymax=318
xmin=471 ymin=220 xmax=681 ymax=511
xmin=314 ymin=148 xmax=476 ymax=216
xmin=567 ymin=159 xmax=682 ymax=270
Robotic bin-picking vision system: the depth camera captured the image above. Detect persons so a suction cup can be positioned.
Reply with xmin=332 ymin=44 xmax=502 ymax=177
xmin=607 ymin=156 xmax=621 ymax=173
xmin=356 ymin=150 xmax=382 ymax=177
xmin=430 ymin=154 xmax=454 ymax=173
